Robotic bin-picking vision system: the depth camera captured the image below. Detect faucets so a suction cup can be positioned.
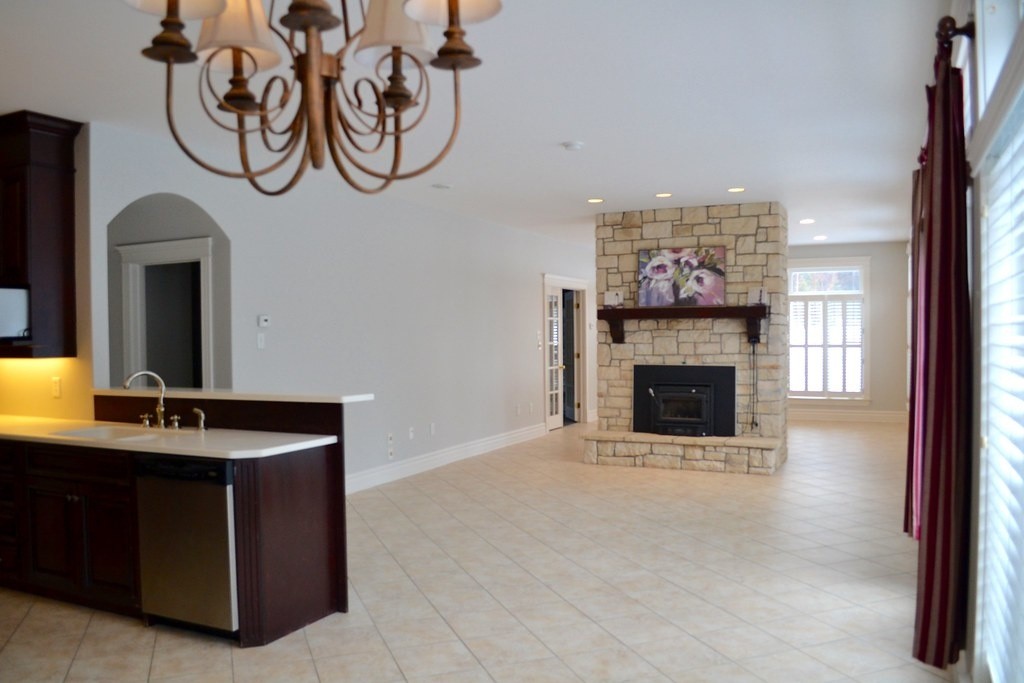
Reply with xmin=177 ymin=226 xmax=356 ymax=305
xmin=123 ymin=368 xmax=167 ymax=429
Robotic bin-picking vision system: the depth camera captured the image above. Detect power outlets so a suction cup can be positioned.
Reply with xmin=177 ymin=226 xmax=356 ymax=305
xmin=387 ymin=433 xmax=392 ymax=443
xmin=408 ymin=426 xmax=414 ymax=439
xmin=387 ymin=447 xmax=393 ymax=459
xmin=51 ymin=376 xmax=60 ymax=397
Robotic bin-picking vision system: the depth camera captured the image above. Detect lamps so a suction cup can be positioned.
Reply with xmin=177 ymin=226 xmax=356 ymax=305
xmin=124 ymin=0 xmax=504 ymax=197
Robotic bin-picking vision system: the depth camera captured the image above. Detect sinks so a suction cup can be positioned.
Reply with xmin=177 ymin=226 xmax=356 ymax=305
xmin=51 ymin=424 xmax=148 ymax=441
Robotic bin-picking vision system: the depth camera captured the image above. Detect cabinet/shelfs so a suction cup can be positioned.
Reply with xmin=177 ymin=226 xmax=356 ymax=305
xmin=0 ymin=109 xmax=84 ymax=358
xmin=26 ymin=444 xmax=139 ymax=592
xmin=0 ymin=438 xmax=31 ymax=585
